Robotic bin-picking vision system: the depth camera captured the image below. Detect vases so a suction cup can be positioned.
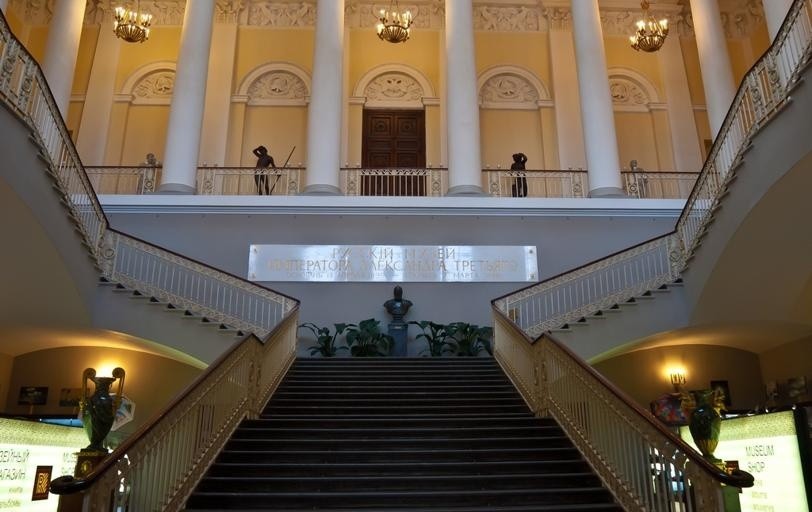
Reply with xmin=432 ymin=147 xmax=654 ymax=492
xmin=76 ymin=366 xmax=126 ymax=453
xmin=687 ymin=387 xmax=724 ymax=464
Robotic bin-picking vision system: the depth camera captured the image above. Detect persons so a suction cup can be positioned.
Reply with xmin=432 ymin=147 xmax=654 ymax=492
xmin=630 ymin=160 xmax=649 ymax=199
xmin=510 ymin=153 xmax=528 ymax=197
xmin=253 ymin=146 xmax=280 ymax=195
xmin=135 ymin=153 xmax=162 ymax=194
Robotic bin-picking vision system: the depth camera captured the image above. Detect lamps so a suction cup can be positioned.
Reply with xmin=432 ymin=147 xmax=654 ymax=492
xmin=668 ymin=367 xmax=688 ymax=392
xmin=627 ymin=1 xmax=670 ymax=54
xmin=110 ymin=1 xmax=156 ymax=46
xmin=373 ymin=0 xmax=421 ymax=44
